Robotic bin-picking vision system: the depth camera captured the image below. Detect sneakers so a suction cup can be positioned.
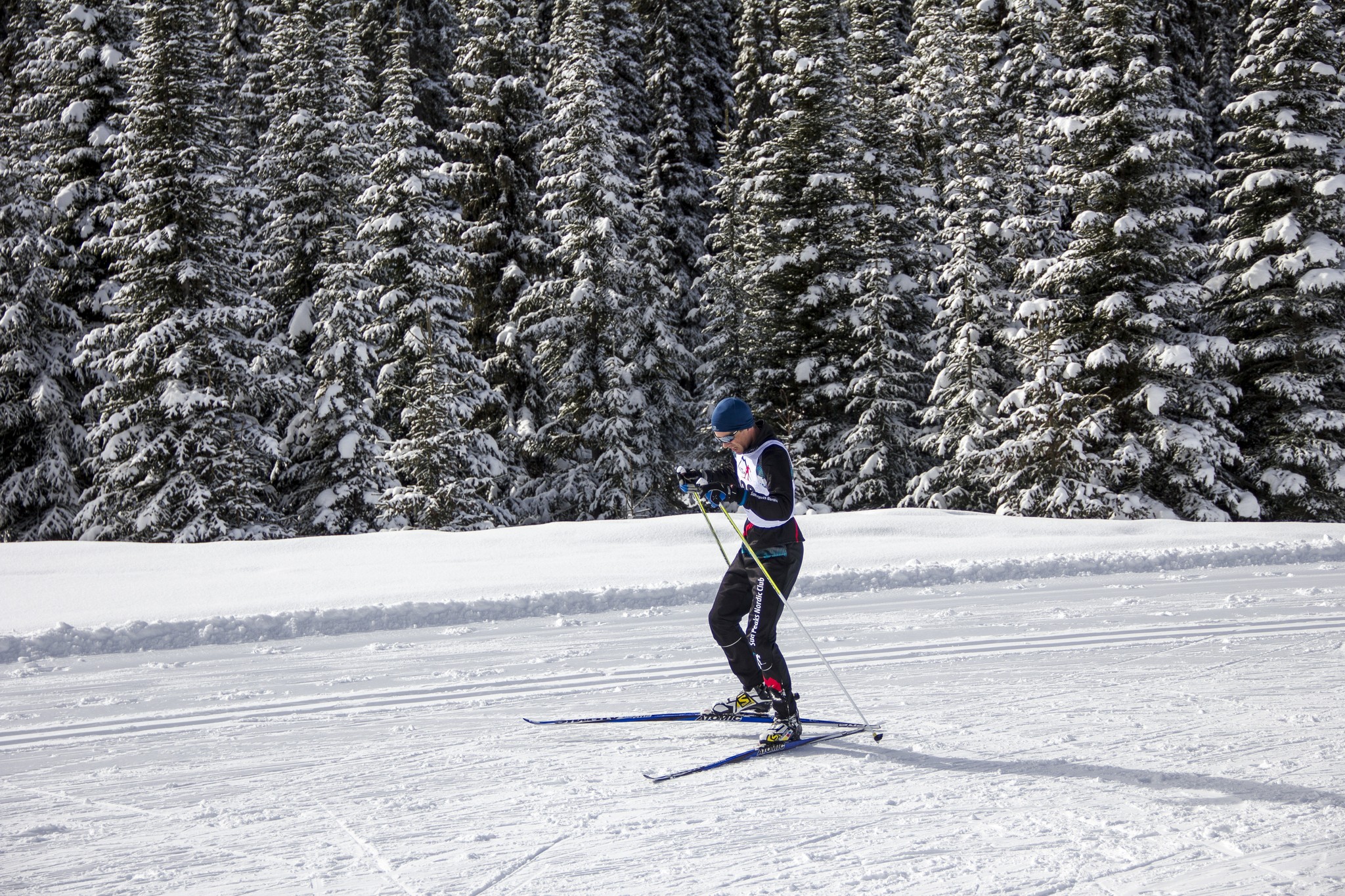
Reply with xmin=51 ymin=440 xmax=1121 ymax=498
xmin=712 ymin=684 xmax=773 ymax=714
xmin=759 ymin=715 xmax=802 ymax=744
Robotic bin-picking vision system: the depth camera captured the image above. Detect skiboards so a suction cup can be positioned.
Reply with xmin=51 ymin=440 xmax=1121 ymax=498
xmin=522 ymin=712 xmax=881 ymax=783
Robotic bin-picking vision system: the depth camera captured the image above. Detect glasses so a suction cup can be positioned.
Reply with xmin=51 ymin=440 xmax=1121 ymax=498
xmin=713 ymin=431 xmax=738 ymax=443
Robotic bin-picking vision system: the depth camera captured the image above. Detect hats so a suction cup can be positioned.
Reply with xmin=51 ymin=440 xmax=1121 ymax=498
xmin=711 ymin=397 xmax=753 ymax=432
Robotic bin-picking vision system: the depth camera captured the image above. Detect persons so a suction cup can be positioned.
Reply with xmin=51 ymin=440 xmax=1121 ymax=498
xmin=676 ymin=397 xmax=805 ymax=746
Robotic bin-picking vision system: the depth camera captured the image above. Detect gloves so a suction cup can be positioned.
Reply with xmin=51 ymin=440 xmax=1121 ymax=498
xmin=677 ymin=468 xmax=703 ymax=493
xmin=699 ymin=482 xmax=732 ymax=508
